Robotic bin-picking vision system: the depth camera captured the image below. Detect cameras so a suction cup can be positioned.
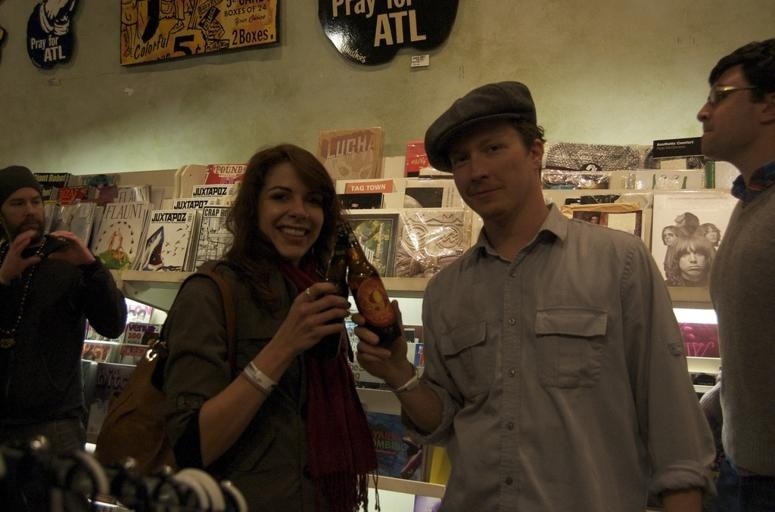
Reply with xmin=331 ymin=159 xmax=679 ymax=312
xmin=19 ymin=231 xmax=63 ymax=258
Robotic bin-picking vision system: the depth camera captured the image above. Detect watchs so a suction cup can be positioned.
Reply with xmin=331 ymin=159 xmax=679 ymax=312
xmin=388 ymin=363 xmax=421 ymax=395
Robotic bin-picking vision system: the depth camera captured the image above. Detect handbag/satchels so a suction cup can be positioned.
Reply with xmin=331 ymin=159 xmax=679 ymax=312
xmin=93 ymin=271 xmax=238 ymax=477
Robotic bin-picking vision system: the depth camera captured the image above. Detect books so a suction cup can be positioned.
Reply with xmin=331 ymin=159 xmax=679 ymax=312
xmin=348 ymin=364 xmax=391 ymax=390
xmin=140 ymin=163 xmax=249 ymax=272
xmin=35 ymin=173 xmax=154 ymax=271
xmin=365 ymin=413 xmax=433 ymax=483
xmin=342 ymin=322 xmax=424 ymax=365
xmin=539 ymin=137 xmax=733 ymax=287
xmin=318 ymin=127 xmax=472 ymax=278
xmin=80 ymin=321 xmax=164 ymax=434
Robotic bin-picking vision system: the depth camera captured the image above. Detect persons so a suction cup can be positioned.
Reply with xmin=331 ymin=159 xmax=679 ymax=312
xmin=153 ymin=143 xmax=378 ymax=512
xmin=700 ymin=223 xmax=720 ymax=245
xmin=352 ymin=82 xmax=719 ymax=512
xmin=675 ymin=213 xmax=699 ymax=235
xmin=82 ymin=344 xmax=96 ymax=361
xmin=0 ymin=166 xmax=126 ymax=450
xmin=662 ymin=226 xmax=681 ymax=246
xmin=699 ymin=39 xmax=775 ymax=512
xmin=665 ymin=235 xmax=716 ymax=287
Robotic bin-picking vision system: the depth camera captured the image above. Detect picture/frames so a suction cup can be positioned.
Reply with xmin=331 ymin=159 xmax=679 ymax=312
xmin=120 ymin=0 xmax=280 ymax=68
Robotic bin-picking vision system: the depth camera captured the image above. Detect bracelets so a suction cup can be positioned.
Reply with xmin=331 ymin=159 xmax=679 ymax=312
xmin=246 ymin=362 xmax=277 ymax=393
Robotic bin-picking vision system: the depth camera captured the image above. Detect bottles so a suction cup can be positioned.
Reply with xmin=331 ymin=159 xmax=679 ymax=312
xmin=335 ymin=221 xmax=401 ymax=348
xmin=303 ymin=231 xmax=349 ymax=360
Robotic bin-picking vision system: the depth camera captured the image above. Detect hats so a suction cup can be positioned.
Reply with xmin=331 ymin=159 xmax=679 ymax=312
xmin=424 ymin=80 xmax=538 ymax=171
xmin=0 ymin=165 xmax=43 ymax=204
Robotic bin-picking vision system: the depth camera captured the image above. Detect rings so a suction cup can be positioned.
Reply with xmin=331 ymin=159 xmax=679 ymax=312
xmin=304 ymin=287 xmax=314 ymax=302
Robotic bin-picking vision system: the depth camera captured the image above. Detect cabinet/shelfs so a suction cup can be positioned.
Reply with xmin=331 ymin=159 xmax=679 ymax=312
xmin=31 ymin=165 xmax=742 ymax=503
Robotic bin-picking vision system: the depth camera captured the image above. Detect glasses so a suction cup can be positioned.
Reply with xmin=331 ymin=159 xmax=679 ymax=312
xmin=708 ymin=83 xmax=758 ymax=103
